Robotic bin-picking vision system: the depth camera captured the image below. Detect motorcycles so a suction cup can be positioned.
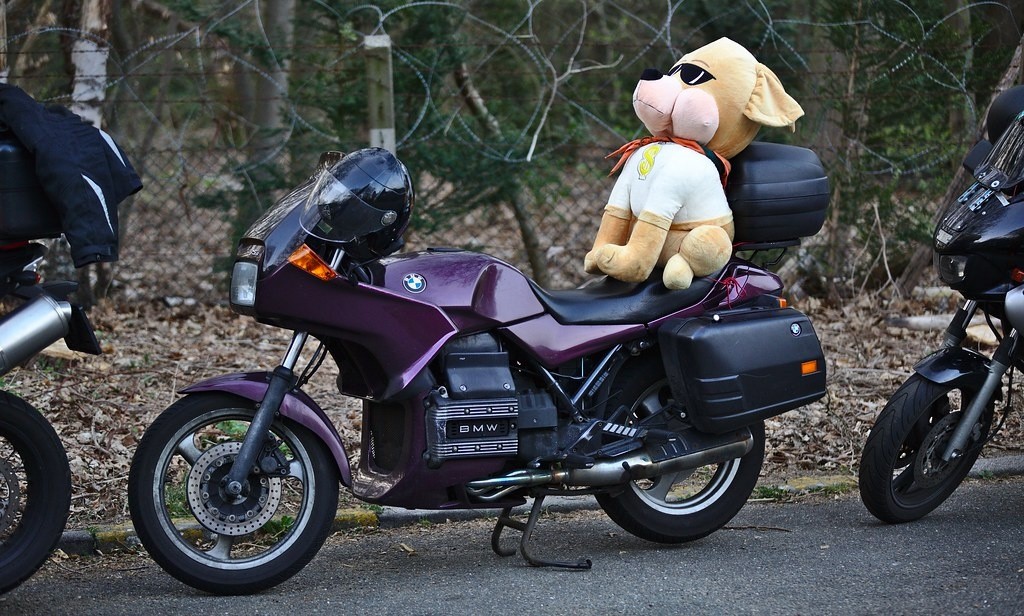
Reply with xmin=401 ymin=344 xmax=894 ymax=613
xmin=0 ymin=232 xmax=107 ymax=608
xmin=857 ymin=81 xmax=1023 ymax=526
xmin=128 ymin=142 xmax=833 ymax=598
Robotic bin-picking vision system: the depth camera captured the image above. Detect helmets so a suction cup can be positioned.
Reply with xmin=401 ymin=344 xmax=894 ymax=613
xmin=986 ymin=85 xmax=1024 ymax=145
xmin=299 ymin=147 xmax=415 ymax=246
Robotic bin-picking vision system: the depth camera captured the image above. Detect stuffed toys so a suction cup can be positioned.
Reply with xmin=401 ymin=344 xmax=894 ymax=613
xmin=584 ymin=39 xmax=804 ymax=291
